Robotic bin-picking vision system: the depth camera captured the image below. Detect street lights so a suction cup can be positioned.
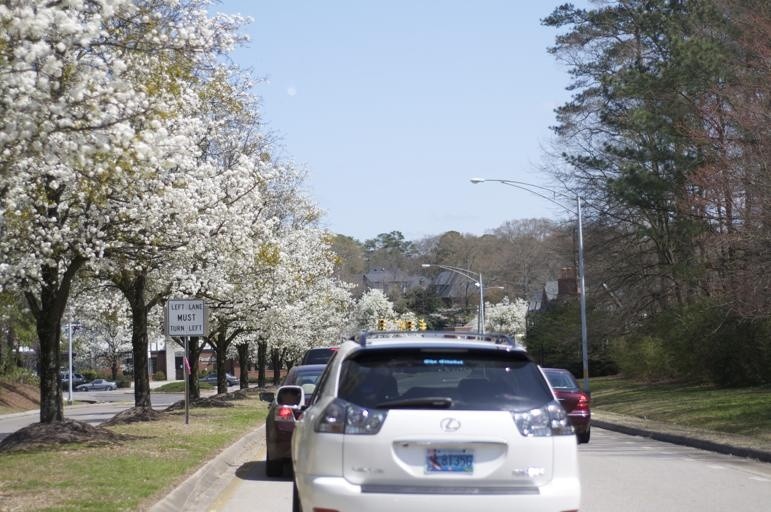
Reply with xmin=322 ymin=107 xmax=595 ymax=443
xmin=471 ymin=178 xmax=591 ymax=400
xmin=67 ymin=317 xmax=74 ymax=400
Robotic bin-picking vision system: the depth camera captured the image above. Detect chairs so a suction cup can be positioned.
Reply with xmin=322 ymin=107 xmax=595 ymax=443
xmin=361 ymin=373 xmax=491 ymax=401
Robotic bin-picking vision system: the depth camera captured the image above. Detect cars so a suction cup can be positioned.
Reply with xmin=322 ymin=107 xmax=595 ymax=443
xmin=541 ymin=367 xmax=589 ymax=442
xmin=303 ymin=349 xmax=337 ymax=365
xmin=257 ymin=364 xmax=330 ymax=477
xmin=422 ymin=263 xmax=485 ymax=335
xmin=276 ymin=332 xmax=580 ymax=511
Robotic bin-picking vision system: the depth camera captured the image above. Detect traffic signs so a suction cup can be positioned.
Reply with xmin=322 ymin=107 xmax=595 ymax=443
xmin=167 ymin=300 xmax=205 ymax=336
